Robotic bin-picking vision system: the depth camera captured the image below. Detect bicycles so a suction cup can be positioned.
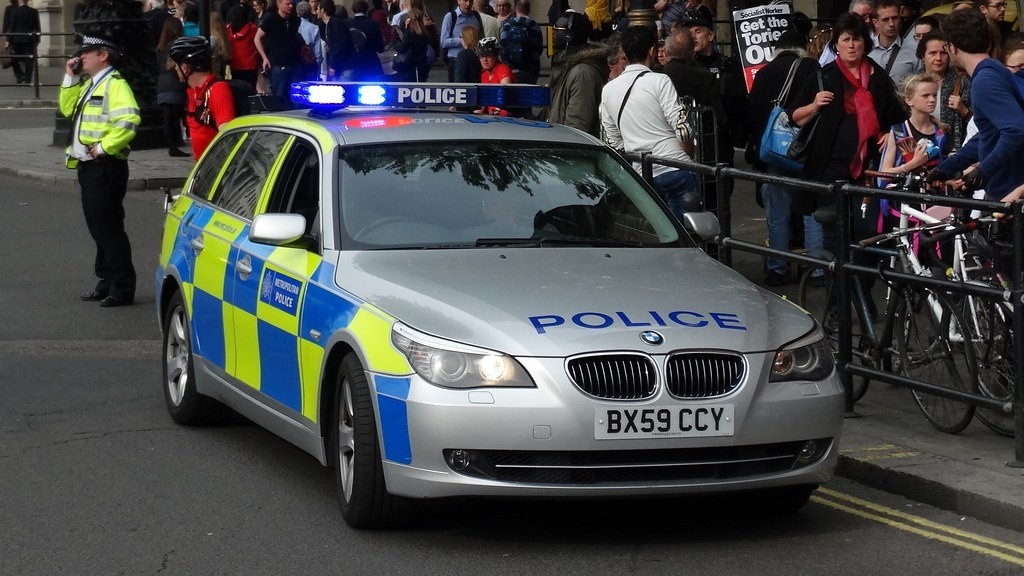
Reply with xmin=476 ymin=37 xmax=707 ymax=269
xmin=797 ymin=167 xmax=1020 ymax=439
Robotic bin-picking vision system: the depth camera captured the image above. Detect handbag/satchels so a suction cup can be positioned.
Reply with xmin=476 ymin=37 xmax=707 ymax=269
xmin=1 ymin=46 xmax=18 ymax=69
xmin=787 ymin=70 xmax=830 ymax=168
xmin=758 ymin=104 xmax=805 ymax=173
xmin=391 ymin=49 xmax=412 ymax=72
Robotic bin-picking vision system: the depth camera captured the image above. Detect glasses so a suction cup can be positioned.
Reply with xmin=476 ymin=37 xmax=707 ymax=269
xmin=988 ymin=3 xmax=1008 ymax=10
xmin=1006 ymin=65 xmax=1021 ymax=72
xmin=552 ymin=25 xmax=567 ymax=33
xmin=942 ymin=43 xmax=949 ymax=52
xmin=913 ymin=35 xmax=923 ymax=40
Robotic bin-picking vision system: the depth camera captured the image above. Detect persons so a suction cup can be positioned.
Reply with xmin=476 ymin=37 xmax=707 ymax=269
xmin=59 ymin=29 xmax=141 ymax=307
xmin=548 ymin=0 xmax=749 ymax=269
xmin=384 ymin=0 xmax=440 ymax=82
xmin=138 ymin=0 xmax=385 ymax=157
xmin=3 ymin=0 xmax=41 ymax=83
xmin=168 ymin=35 xmax=235 ymax=165
xmin=744 ymin=0 xmax=1024 ymax=388
xmin=441 ymin=0 xmax=543 ymax=119
xmin=464 ymin=171 xmax=559 ymax=232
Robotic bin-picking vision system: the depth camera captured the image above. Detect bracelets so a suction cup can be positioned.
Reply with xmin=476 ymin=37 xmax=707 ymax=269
xmin=901 ymin=164 xmax=908 ymax=174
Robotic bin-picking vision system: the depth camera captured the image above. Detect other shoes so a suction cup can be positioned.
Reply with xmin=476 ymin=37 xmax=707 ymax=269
xmin=18 ymin=75 xmax=25 ymax=83
xmin=169 ymin=149 xmax=191 ymax=156
xmin=864 ymin=295 xmax=877 ymax=320
xmin=809 ymin=276 xmax=825 ymax=287
xmin=99 ymin=295 xmax=133 ymax=306
xmin=82 ymin=290 xmax=106 ymax=300
xmin=765 ymin=273 xmax=784 ymax=286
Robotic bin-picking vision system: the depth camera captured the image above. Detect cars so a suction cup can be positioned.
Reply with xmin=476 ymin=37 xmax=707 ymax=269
xmin=158 ymin=81 xmax=848 ymax=533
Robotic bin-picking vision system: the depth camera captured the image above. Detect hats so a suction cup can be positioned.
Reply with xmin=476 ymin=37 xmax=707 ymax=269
xmin=76 ymin=32 xmax=119 ymax=53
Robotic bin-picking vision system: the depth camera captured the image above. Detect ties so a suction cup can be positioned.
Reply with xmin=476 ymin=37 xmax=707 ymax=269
xmin=65 ymin=81 xmax=93 ymax=147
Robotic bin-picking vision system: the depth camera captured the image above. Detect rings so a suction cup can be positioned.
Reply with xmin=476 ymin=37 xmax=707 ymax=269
xmin=951 ymin=102 xmax=954 ymax=105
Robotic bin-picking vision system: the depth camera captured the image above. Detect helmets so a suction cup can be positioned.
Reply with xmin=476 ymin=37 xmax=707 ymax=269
xmin=476 ymin=37 xmax=502 ymax=55
xmin=169 ymin=35 xmax=212 ymax=67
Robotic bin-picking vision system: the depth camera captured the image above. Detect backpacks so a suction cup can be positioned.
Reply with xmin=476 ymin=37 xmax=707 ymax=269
xmin=499 ymin=17 xmax=536 ymax=69
